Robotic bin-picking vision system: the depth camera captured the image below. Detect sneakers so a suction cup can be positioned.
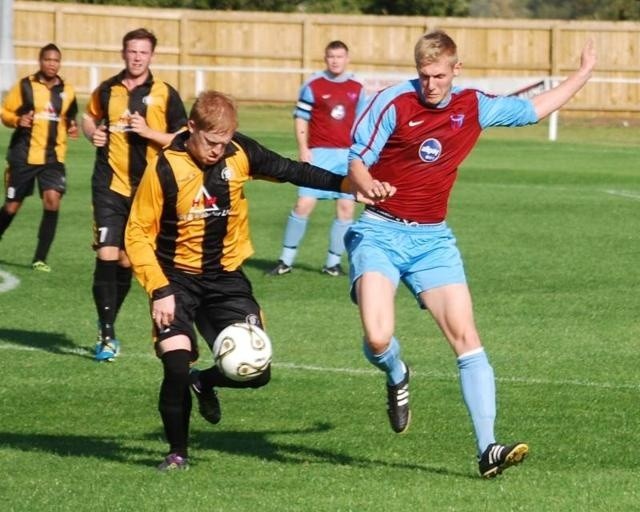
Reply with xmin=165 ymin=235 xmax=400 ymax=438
xmin=267 ymin=260 xmax=291 ymax=274
xmin=387 ymin=360 xmax=411 ymax=434
xmin=95 ymin=320 xmax=120 ymax=361
xmin=477 ymin=443 xmax=528 ymax=478
xmin=158 ymin=454 xmax=189 ymax=471
xmin=322 ymin=264 xmax=346 ymax=276
xmin=31 ymin=260 xmax=50 ymax=272
xmin=189 ymin=368 xmax=220 ymax=424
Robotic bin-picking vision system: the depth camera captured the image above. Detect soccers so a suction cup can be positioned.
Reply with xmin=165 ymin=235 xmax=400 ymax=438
xmin=212 ymin=320 xmax=271 ymax=383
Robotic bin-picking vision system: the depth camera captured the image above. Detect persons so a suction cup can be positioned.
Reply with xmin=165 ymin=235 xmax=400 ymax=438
xmin=76 ymin=27 xmax=188 ymax=366
xmin=120 ymin=84 xmax=395 ymax=474
xmin=265 ymin=40 xmax=376 ymax=279
xmin=344 ymin=29 xmax=600 ymax=480
xmin=0 ymin=42 xmax=79 ymax=272
